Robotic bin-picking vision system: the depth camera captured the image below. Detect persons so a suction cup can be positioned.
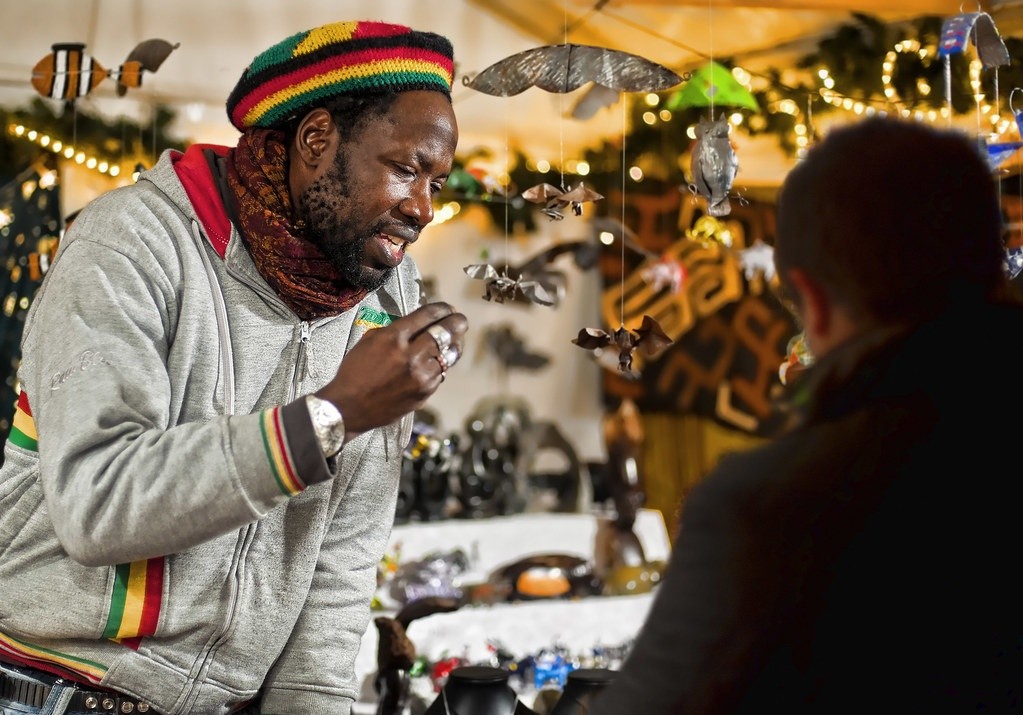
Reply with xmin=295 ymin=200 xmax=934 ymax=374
xmin=588 ymin=118 xmax=1023 ymax=715
xmin=0 ymin=22 xmax=469 ymax=715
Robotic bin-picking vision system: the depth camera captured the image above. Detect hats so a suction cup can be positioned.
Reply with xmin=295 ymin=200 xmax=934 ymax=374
xmin=227 ymin=22 xmax=454 ymax=132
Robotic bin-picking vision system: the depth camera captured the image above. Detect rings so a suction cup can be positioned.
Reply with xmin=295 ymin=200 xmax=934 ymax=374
xmin=427 ymin=324 xmax=457 ymax=383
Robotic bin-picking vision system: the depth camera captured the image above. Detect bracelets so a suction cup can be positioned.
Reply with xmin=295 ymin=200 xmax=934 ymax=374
xmin=305 ymin=395 xmax=344 ymax=458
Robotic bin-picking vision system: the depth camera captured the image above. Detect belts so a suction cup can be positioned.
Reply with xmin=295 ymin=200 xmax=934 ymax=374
xmin=0 ymin=672 xmax=159 ymax=715
